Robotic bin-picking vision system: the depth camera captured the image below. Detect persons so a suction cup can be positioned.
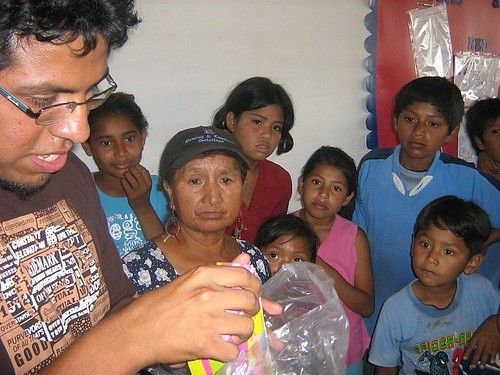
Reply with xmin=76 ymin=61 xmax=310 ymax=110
xmin=84 ymin=91 xmax=170 ymax=259
xmin=351 ymin=75 xmax=500 ymax=315
xmin=367 ymin=198 xmax=500 ymax=374
xmin=464 ymin=97 xmax=500 ymax=193
xmin=300 ymin=145 xmax=376 ymax=374
xmin=1 ymin=0 xmax=287 ymax=375
xmin=251 ymin=212 xmax=335 ymax=375
xmin=214 ymin=77 xmax=296 ymax=246
xmin=118 ymin=124 xmax=275 ymax=375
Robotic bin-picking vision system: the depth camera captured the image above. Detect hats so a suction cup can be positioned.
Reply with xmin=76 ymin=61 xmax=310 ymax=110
xmin=159 ymin=126 xmax=251 ymax=184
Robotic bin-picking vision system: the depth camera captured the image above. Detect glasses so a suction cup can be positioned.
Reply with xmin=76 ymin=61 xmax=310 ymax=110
xmin=0 ymin=75 xmax=118 ymax=126
